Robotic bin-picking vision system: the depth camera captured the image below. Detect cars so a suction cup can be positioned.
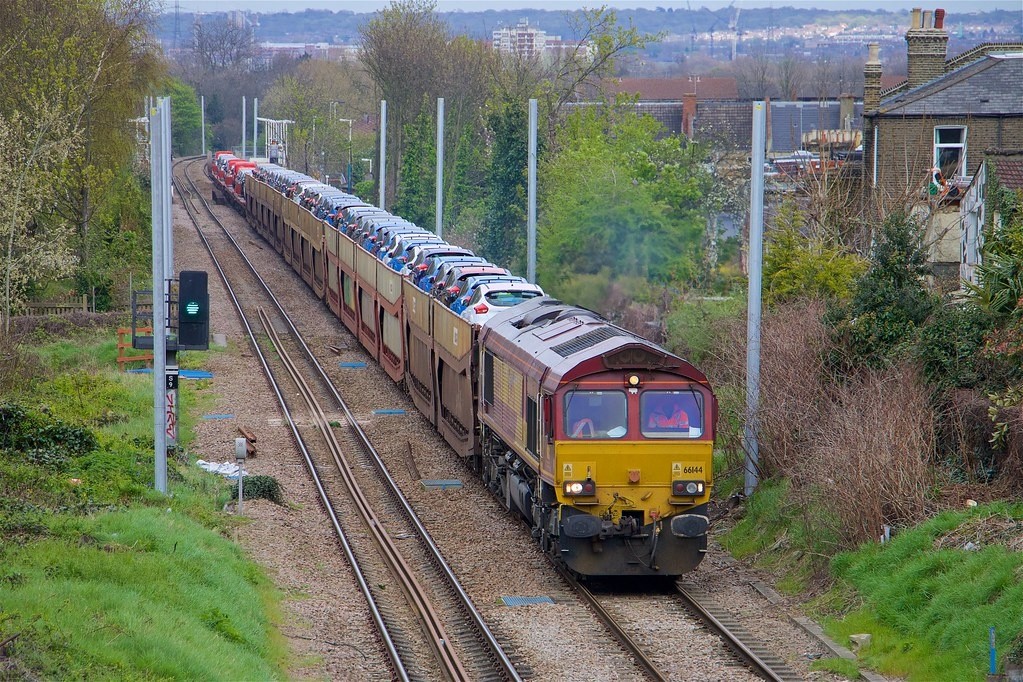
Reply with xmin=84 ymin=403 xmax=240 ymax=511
xmin=210 ymin=152 xmax=512 ymax=308
xmin=449 ymin=276 xmax=530 ymax=315
xmin=459 ymin=282 xmax=547 ymax=327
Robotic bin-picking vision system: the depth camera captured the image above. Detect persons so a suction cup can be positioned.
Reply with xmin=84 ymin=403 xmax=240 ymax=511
xmin=648 ymin=397 xmax=689 ymax=428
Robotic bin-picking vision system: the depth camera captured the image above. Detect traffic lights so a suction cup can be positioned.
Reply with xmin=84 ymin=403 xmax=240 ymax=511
xmin=178 ymin=271 xmax=210 ymax=351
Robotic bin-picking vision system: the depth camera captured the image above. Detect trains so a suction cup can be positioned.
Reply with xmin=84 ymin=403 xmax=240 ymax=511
xmin=205 ymin=150 xmax=717 ymax=583
